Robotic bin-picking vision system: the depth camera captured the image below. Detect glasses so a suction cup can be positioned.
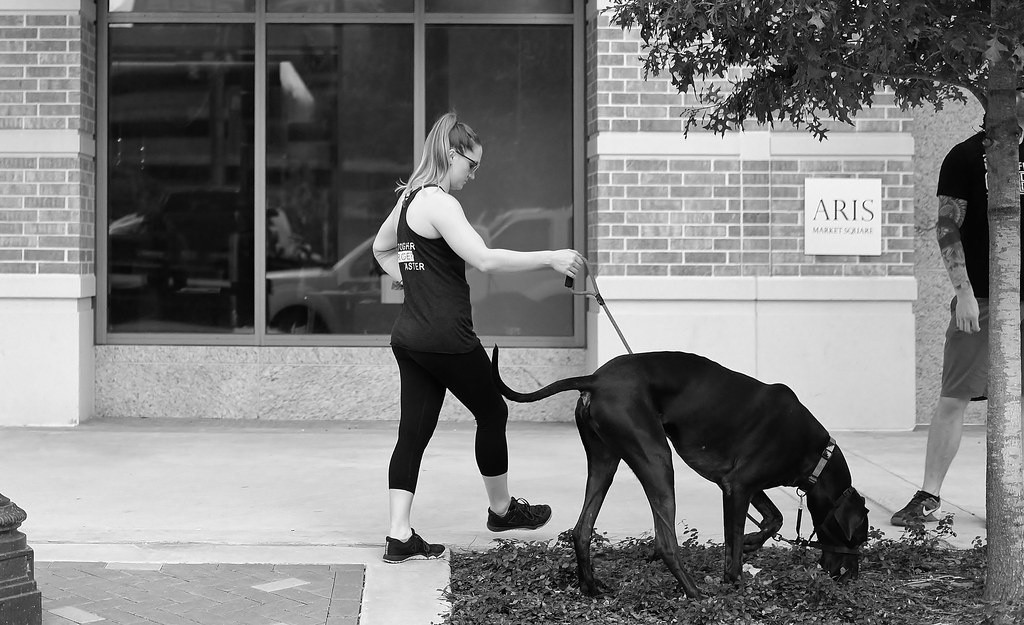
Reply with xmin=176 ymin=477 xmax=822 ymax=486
xmin=456 ymin=150 xmax=480 ymax=173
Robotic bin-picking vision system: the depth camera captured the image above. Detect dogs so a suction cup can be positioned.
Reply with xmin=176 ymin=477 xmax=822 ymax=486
xmin=489 ymin=342 xmax=875 ymax=606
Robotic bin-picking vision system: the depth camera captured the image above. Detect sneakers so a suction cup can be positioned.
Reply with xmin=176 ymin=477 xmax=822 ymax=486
xmin=890 ymin=490 xmax=942 ymax=526
xmin=487 ymin=497 xmax=553 ymax=533
xmin=383 ymin=527 xmax=445 ymax=564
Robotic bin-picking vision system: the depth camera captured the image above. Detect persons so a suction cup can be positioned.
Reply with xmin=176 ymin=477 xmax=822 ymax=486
xmin=373 ymin=108 xmax=585 ymax=563
xmin=890 ymin=91 xmax=1024 ymax=525
